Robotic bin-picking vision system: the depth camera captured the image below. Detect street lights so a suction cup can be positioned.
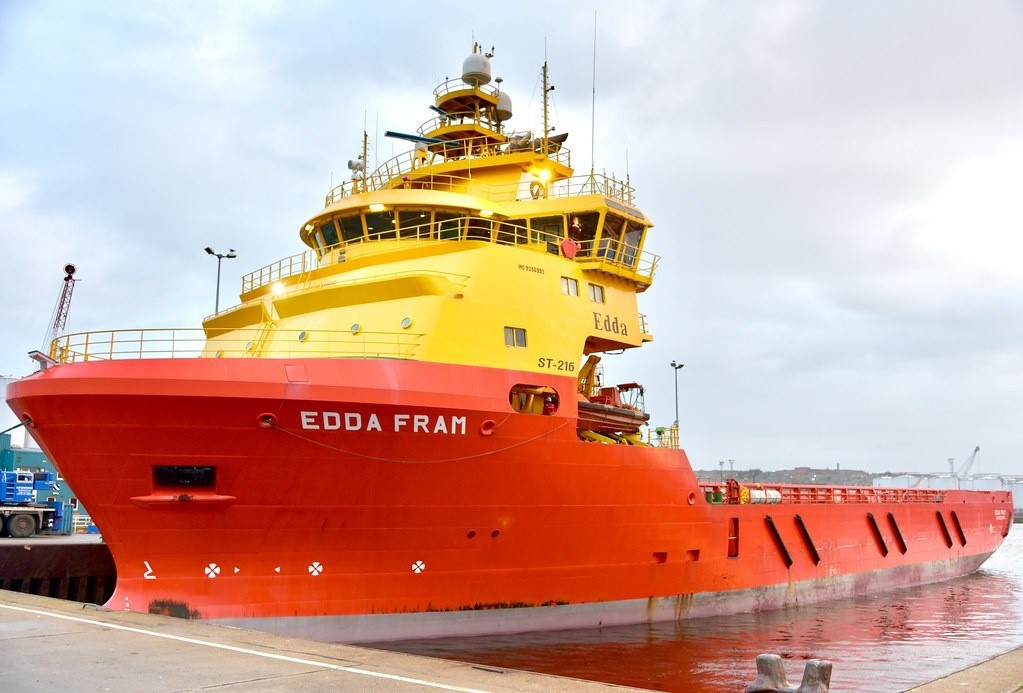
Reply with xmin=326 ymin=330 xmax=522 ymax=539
xmin=670 ymin=360 xmax=685 ymax=428
xmin=203 ymin=247 xmax=237 ymax=316
xmin=719 ymin=462 xmax=724 ymax=484
xmin=728 ymin=459 xmax=735 ymax=478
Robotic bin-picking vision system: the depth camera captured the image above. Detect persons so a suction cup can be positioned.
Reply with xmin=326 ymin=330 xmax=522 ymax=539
xmin=542 ymin=397 xmax=556 ymax=416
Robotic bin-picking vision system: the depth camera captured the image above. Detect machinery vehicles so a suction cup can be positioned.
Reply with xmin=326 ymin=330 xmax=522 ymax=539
xmin=0 ymin=263 xmax=81 ymax=539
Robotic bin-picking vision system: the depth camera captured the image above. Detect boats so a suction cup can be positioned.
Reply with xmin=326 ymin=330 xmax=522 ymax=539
xmin=3 ymin=7 xmax=1017 ymax=644
xmin=542 ymin=385 xmax=651 ymax=432
